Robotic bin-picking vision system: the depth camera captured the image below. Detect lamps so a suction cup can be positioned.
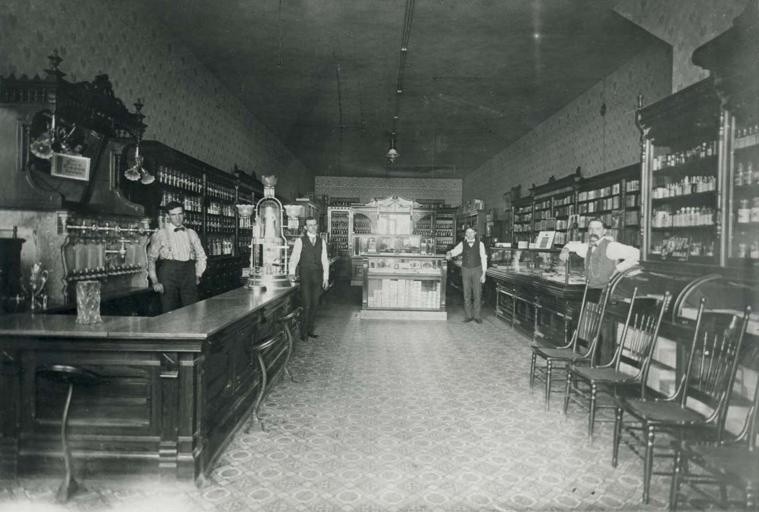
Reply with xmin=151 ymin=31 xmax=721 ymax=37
xmin=386 ymin=133 xmax=400 ymax=159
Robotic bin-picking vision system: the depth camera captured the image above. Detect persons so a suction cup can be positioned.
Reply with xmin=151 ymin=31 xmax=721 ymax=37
xmin=559 ymin=219 xmax=640 ymax=303
xmin=288 ymin=216 xmax=329 ymax=341
xmin=148 ymin=202 xmax=207 ymax=313
xmin=446 ymin=228 xmax=487 ymax=323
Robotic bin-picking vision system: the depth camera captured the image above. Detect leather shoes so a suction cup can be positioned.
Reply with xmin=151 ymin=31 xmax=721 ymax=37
xmin=464 ymin=317 xmax=482 ymax=324
xmin=301 ymin=333 xmax=317 ymax=341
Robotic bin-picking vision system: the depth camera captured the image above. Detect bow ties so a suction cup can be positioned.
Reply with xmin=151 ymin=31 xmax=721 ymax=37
xmin=174 ymin=227 xmax=184 ymax=231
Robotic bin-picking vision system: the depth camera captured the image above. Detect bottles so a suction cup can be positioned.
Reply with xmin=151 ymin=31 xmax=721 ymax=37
xmin=512 ymin=176 xmax=640 ymax=248
xmin=651 ymin=124 xmax=758 ymax=260
xmin=156 ymin=164 xmax=252 ymax=258
xmin=328 ymin=204 xmax=459 ymax=255
xmin=62 ymin=226 xmax=106 ymax=273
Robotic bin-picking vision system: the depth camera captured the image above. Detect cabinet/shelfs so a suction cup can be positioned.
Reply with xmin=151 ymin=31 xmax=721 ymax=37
xmin=120 ymin=139 xmax=263 ymax=258
xmin=511 ymin=162 xmax=642 ymax=248
xmin=327 ymin=206 xmax=456 ymax=286
xmin=635 ymin=77 xmax=759 ymax=282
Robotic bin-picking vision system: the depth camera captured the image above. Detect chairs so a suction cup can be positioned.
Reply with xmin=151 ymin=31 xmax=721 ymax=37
xmin=670 ymin=379 xmax=759 ymax=512
xmin=610 ymin=297 xmax=752 ymax=504
xmin=563 ymin=287 xmax=669 ymax=443
xmin=529 ymin=278 xmax=612 ymax=408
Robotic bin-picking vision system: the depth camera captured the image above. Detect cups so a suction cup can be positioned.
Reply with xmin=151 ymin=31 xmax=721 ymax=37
xmin=74 ymin=280 xmax=104 ymax=324
